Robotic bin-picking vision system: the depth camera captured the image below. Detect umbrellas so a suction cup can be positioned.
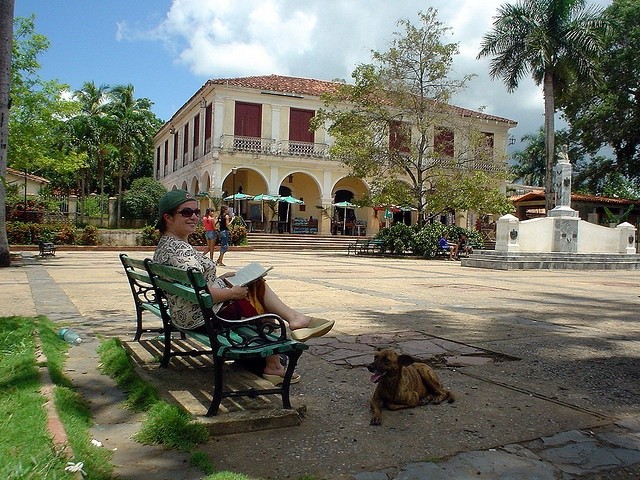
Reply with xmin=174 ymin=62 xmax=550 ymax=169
xmin=251 ymin=192 xmax=277 ymax=223
xmin=277 ymin=195 xmax=305 ymax=224
xmin=223 ymin=192 xmax=255 ymax=217
xmin=331 ymin=201 xmax=361 ymax=231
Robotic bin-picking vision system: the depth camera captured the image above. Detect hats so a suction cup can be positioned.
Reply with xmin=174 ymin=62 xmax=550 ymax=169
xmin=154 ymin=189 xmax=197 ymax=231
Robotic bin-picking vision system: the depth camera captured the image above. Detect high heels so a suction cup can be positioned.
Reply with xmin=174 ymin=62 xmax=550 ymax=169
xmin=292 ymin=316 xmax=335 ymax=342
xmin=263 ymin=373 xmax=301 ymax=387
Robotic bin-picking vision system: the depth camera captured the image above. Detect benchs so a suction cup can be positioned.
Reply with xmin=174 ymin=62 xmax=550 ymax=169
xmin=116 ymin=251 xmax=311 ymax=416
xmin=35 ymin=237 xmax=56 ymax=256
xmin=345 ymin=238 xmax=389 ymax=258
xmin=438 ymin=239 xmax=471 ymax=260
xmin=308 ymin=220 xmax=318 ymax=233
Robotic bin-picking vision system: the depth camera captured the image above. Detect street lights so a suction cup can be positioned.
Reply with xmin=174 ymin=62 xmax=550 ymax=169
xmin=232 ymin=167 xmax=238 ymax=214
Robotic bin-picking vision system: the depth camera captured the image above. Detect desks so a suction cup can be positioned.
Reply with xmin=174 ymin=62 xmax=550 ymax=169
xmin=269 ymin=220 xmax=284 ymax=233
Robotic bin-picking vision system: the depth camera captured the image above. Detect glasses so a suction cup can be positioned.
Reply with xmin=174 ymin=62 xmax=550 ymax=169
xmin=177 ymin=207 xmax=201 ymax=218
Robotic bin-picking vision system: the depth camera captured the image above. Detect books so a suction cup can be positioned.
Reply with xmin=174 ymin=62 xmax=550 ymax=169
xmin=222 ymin=261 xmax=274 ymax=289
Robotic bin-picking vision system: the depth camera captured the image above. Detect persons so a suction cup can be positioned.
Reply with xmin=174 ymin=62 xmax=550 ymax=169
xmin=151 ymin=188 xmax=335 ymax=386
xmin=438 ymin=229 xmax=461 ymax=262
xmin=457 ymin=232 xmax=474 ymax=258
xmin=215 ymin=204 xmax=236 ymax=266
xmin=201 ymin=207 xmax=218 ymax=262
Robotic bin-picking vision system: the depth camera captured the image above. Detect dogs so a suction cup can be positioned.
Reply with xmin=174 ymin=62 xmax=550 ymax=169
xmin=366 ymin=347 xmax=455 ymax=426
